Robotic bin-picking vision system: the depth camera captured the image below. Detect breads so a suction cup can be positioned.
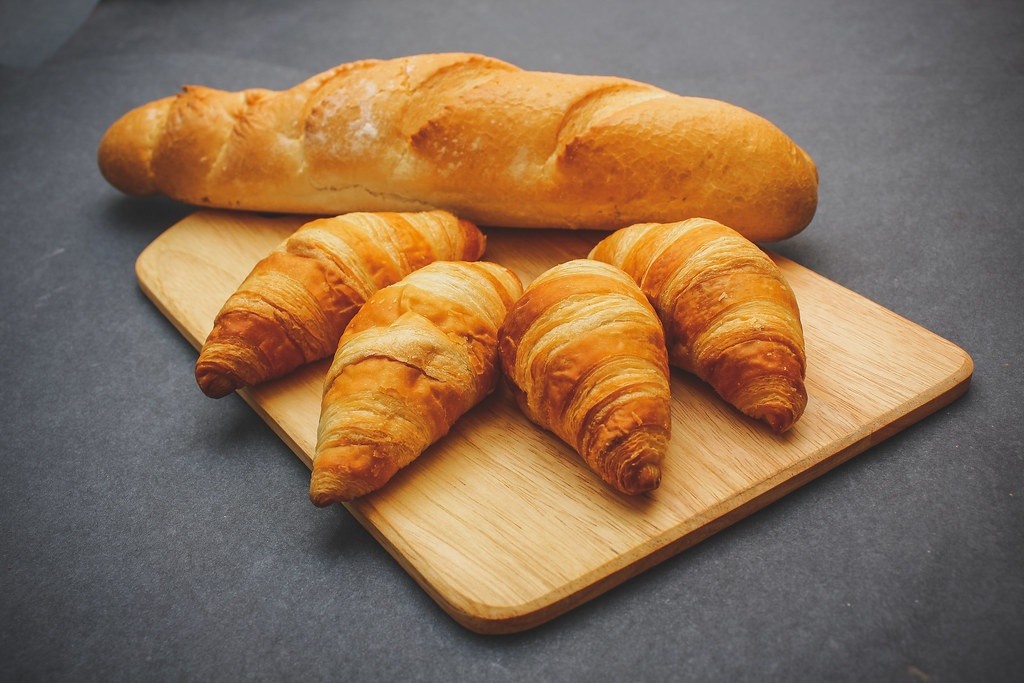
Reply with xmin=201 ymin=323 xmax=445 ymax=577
xmin=100 ymin=53 xmax=816 ymax=505
